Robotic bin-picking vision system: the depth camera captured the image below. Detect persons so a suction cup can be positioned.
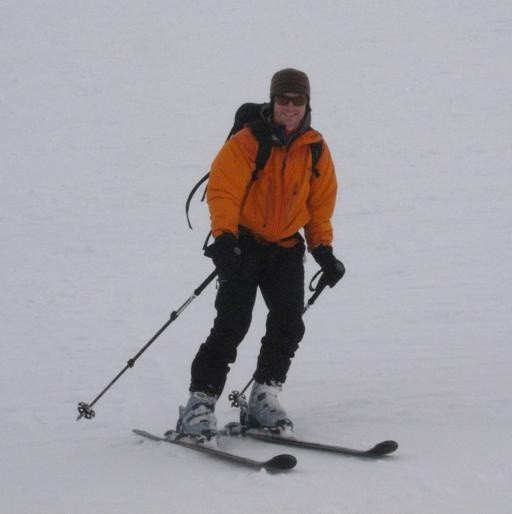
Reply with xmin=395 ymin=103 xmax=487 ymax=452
xmin=180 ymin=68 xmax=345 ymax=440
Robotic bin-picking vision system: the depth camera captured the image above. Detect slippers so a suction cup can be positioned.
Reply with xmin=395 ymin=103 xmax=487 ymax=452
xmin=224 ymin=102 xmax=323 ymax=181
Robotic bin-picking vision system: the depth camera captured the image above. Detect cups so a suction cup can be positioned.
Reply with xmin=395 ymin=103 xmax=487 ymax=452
xmin=273 ymin=93 xmax=308 ymax=108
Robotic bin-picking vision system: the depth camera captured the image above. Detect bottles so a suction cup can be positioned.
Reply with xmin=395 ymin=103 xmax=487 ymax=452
xmin=269 ymin=67 xmax=310 ymax=93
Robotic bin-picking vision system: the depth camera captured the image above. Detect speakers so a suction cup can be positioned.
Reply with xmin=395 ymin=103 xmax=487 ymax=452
xmin=242 ymin=383 xmax=296 ymax=430
xmin=180 ymin=390 xmax=219 ymax=436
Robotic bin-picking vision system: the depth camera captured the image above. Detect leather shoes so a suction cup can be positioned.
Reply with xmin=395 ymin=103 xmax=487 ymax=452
xmin=310 ymin=246 xmax=345 ymax=287
xmin=211 ymin=232 xmax=238 ymax=263
